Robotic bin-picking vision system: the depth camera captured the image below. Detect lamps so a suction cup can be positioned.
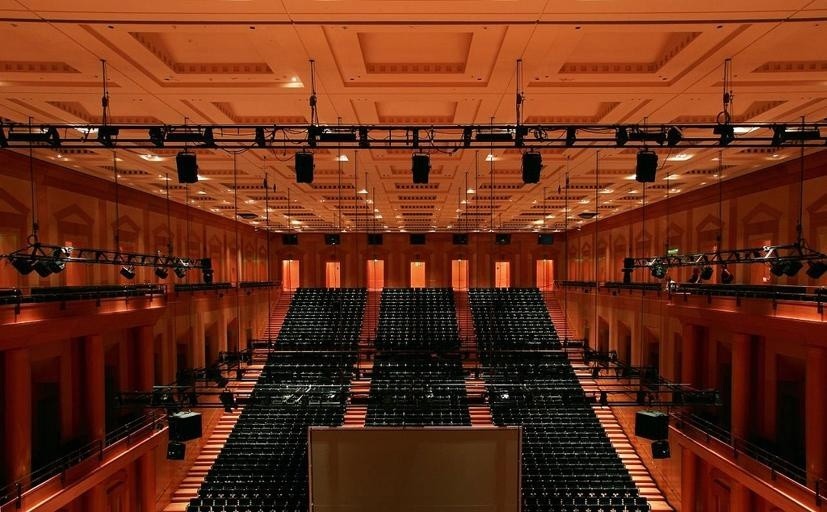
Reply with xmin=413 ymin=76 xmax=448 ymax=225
xmin=294 ymin=152 xmax=314 ymax=183
xmin=13 ymin=258 xmax=65 ymax=277
xmin=175 ymin=152 xmax=200 ymax=183
xmin=521 ymin=151 xmax=542 ymax=184
xmin=635 ymin=152 xmax=659 ymax=183
xmin=120 ymin=266 xmax=186 ymax=279
xmin=649 ymin=260 xmax=827 ymax=284
xmin=411 ymin=152 xmax=430 ymax=183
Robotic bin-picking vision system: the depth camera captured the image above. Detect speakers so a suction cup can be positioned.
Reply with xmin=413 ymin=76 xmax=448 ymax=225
xmin=635 ymin=411 xmax=669 ymax=440
xmin=170 ymin=411 xmax=202 ymax=440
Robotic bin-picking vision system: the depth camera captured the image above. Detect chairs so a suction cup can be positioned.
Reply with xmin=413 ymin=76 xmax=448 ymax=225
xmin=364 ymin=287 xmax=471 ymax=426
xmin=186 ymin=288 xmax=370 ymax=512
xmin=467 ymin=288 xmax=651 ymax=511
